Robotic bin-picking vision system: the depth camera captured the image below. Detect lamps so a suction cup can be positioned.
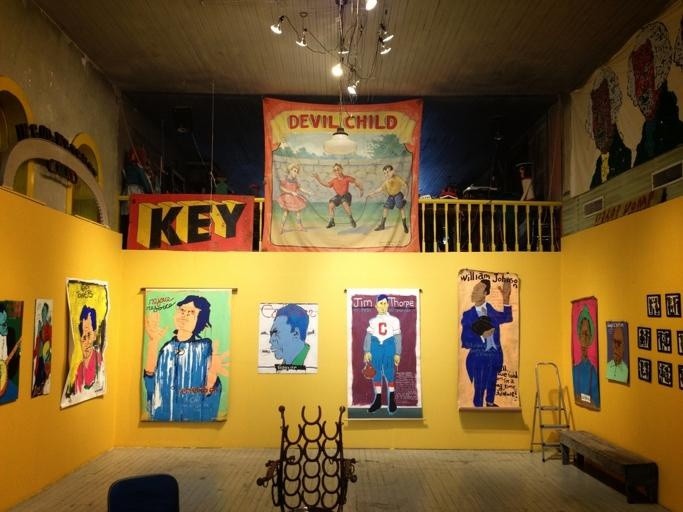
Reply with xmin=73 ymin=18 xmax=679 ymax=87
xmin=269 ymin=0 xmax=396 ymax=97
xmin=322 ymin=1 xmax=358 ymax=155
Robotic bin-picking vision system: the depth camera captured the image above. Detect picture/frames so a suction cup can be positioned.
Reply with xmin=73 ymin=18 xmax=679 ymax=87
xmin=677 ymin=331 xmax=683 ymax=356
xmin=656 ymin=361 xmax=673 ymax=386
xmin=638 ymin=326 xmax=652 ymax=350
xmin=657 ymin=329 xmax=672 ymax=352
xmin=637 ymin=357 xmax=652 ymax=383
xmin=664 ymin=293 xmax=681 ymax=317
xmin=677 ymin=364 xmax=683 ymax=390
xmin=647 ymin=294 xmax=662 ymax=317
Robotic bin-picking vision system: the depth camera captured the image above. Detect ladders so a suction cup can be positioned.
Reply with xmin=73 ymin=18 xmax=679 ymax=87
xmin=530 ymin=362 xmax=570 ymax=463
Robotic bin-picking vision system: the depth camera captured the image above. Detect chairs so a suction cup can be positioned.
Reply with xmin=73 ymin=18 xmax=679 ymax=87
xmin=106 ymin=472 xmax=180 ymax=512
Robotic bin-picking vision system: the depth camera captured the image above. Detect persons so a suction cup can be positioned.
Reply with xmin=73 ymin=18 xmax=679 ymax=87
xmin=314 ymin=162 xmax=367 ymax=237
xmin=608 ymin=325 xmax=626 ymax=382
xmin=146 ymin=295 xmax=227 ymax=421
xmin=516 ymin=157 xmax=542 ymax=251
xmin=33 ymin=300 xmax=55 ymax=398
xmin=576 ymin=308 xmax=599 ymax=405
xmin=371 ymin=162 xmax=410 ymax=239
xmin=269 ymin=303 xmax=311 ymax=371
xmin=585 ymin=68 xmax=633 ymax=190
xmin=74 ymin=313 xmax=108 ymax=395
xmin=461 ymin=279 xmax=515 ymax=413
xmin=362 ymin=296 xmax=404 ymax=417
xmin=0 ymin=305 xmax=22 ymax=407
xmin=118 ymin=142 xmax=155 ymax=219
xmin=622 ymin=21 xmax=683 ymax=154
xmin=274 ymin=159 xmax=310 ymax=237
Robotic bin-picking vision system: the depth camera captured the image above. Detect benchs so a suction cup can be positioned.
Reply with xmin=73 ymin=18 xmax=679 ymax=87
xmin=560 ymin=430 xmax=659 ymax=505
